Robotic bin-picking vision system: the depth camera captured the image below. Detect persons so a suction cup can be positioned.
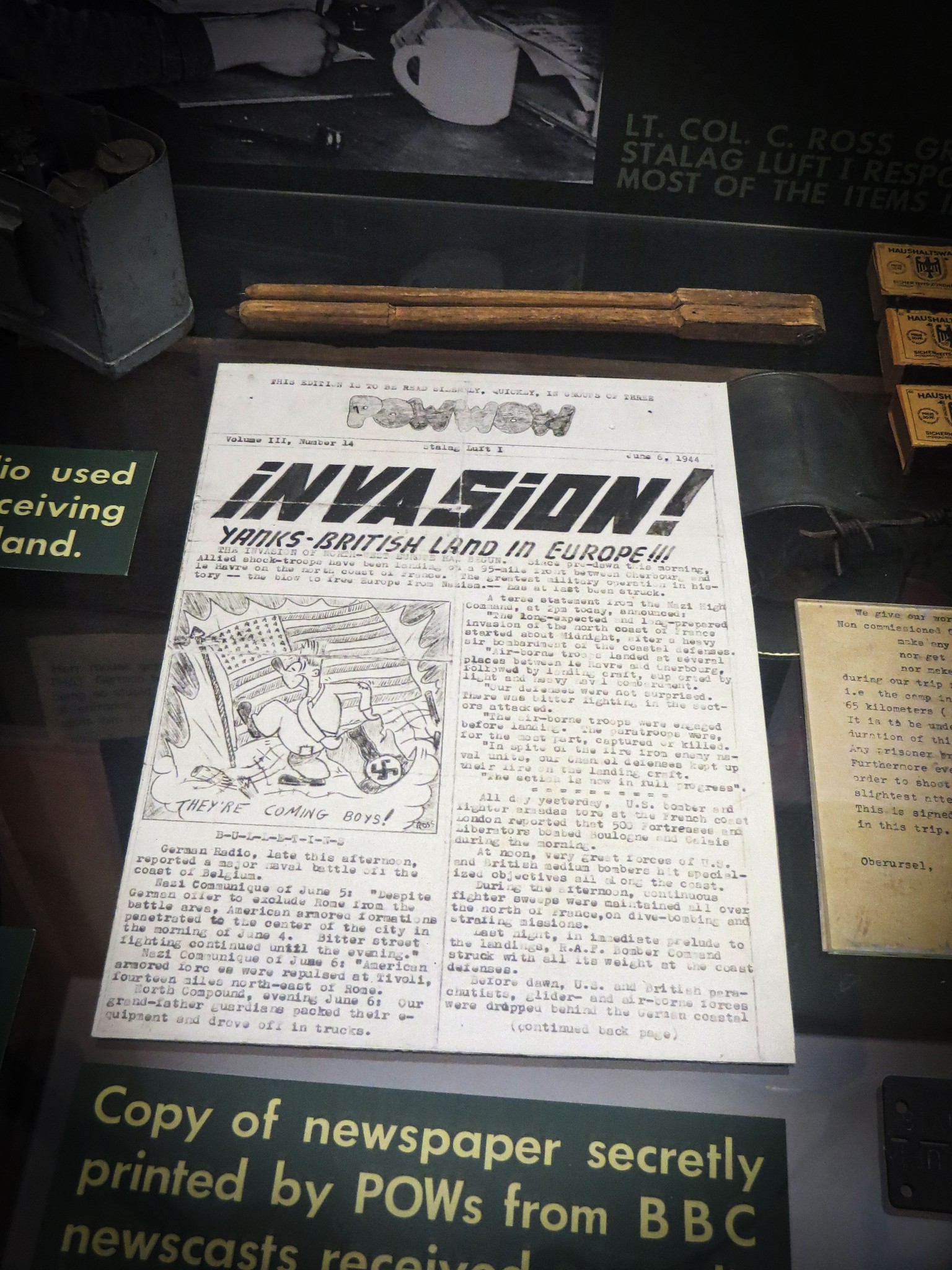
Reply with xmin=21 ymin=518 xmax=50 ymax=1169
xmin=1 ymin=0 xmax=343 ymax=94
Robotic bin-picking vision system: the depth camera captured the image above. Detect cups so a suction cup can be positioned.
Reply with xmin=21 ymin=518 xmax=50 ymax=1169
xmin=392 ymin=28 xmax=521 ymax=127
